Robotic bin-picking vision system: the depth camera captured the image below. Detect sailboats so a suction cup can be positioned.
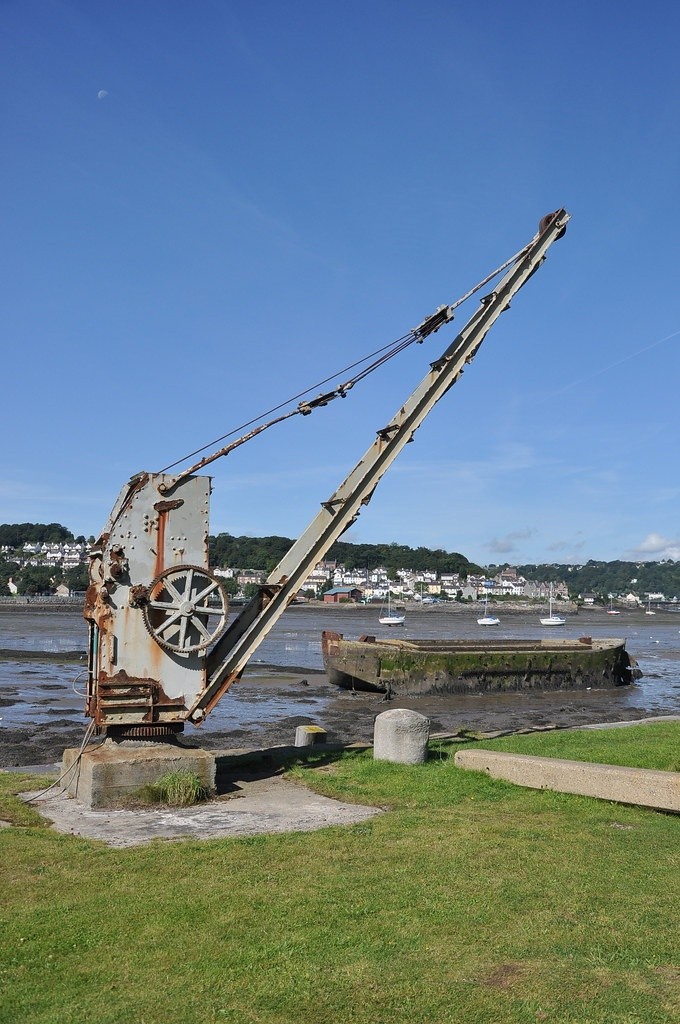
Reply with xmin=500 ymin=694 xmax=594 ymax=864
xmin=645 ymin=600 xmax=655 ymax=615
xmin=540 ymin=583 xmax=566 ymax=626
xmin=377 ymin=585 xmax=405 ymax=627
xmin=476 ymin=588 xmax=500 ymax=626
xmin=602 ymin=595 xmax=620 ymax=615
